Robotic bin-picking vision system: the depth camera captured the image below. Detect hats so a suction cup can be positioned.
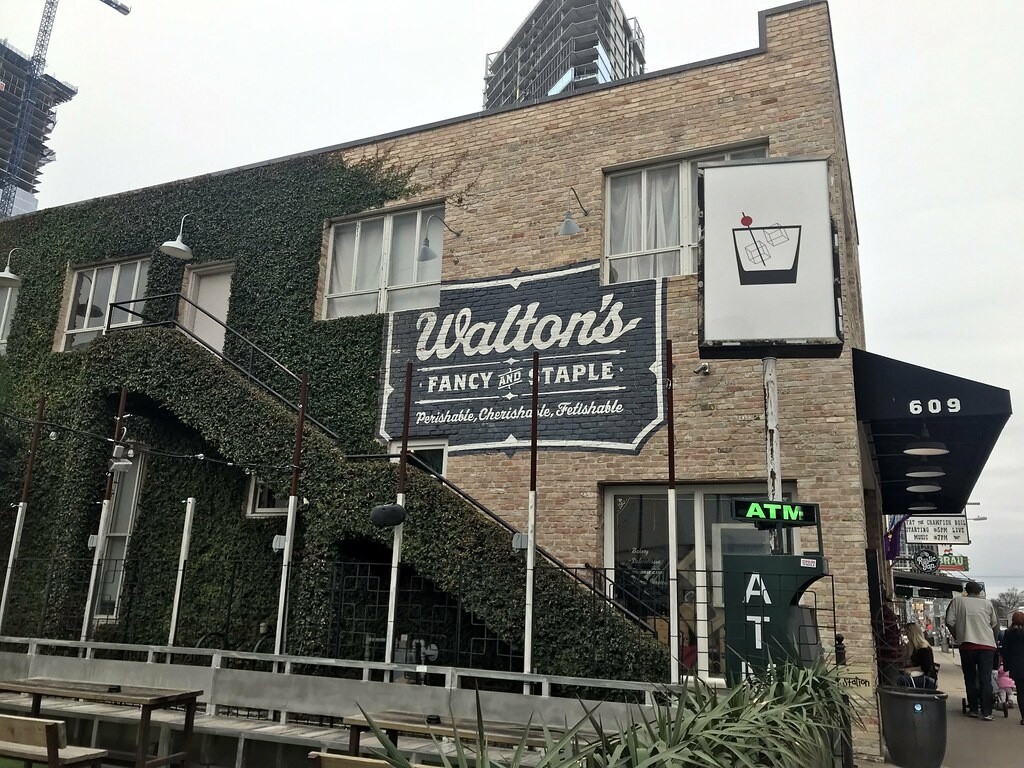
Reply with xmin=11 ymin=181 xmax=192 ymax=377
xmin=1012 ymin=612 xmax=1024 ymax=624
xmin=964 ymin=582 xmax=982 ymax=590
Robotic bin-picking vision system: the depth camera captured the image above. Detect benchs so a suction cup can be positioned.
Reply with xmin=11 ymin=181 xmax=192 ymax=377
xmin=0 ymin=634 xmax=735 ymax=768
xmin=0 ymin=714 xmax=109 ymax=768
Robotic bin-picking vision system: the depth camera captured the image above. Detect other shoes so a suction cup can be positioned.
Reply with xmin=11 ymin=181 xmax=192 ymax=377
xmin=1020 ymin=720 xmax=1024 ymax=725
xmin=1008 ymin=700 xmax=1014 ymax=709
xmin=1000 ymin=708 xmax=1004 ymax=711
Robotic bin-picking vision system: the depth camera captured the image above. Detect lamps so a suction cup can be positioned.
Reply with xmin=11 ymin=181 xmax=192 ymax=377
xmin=158 ymin=212 xmax=196 ymax=261
xmin=557 ymin=187 xmax=588 ymax=236
xmin=0 ymin=247 xmax=37 ymax=287
xmin=878 ymin=478 xmax=943 ymax=493
xmin=868 ymin=433 xmax=950 ymax=456
xmin=417 ymin=214 xmax=461 ymax=262
xmin=882 ymin=492 xmax=938 ymax=511
xmin=873 ymin=457 xmax=946 ymax=478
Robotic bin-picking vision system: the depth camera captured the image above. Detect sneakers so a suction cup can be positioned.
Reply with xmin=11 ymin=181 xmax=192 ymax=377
xmin=967 ymin=710 xmax=979 ymax=718
xmin=983 ymin=714 xmax=995 ymax=721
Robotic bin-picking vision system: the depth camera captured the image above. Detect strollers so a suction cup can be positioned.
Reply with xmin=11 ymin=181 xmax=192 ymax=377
xmin=962 ymin=641 xmax=1014 ymax=718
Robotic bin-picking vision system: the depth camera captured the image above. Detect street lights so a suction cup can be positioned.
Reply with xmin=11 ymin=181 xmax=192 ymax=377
xmin=999 ymin=607 xmax=1007 ymax=619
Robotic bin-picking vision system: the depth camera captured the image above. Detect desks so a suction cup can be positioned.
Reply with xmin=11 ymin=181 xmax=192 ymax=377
xmin=0 ymin=677 xmax=204 ymax=768
xmin=342 ymin=708 xmax=630 ymax=768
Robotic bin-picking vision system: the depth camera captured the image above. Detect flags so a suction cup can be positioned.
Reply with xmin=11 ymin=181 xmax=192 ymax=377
xmin=883 ymin=515 xmax=907 ymax=561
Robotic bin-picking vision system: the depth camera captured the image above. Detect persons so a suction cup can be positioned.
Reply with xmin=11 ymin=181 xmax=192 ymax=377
xmin=898 ymin=622 xmax=937 ymax=690
xmin=1002 ymin=611 xmax=1024 ymax=726
xmin=945 ymin=581 xmax=1000 ymax=721
xmin=915 ymin=614 xmax=955 ymax=655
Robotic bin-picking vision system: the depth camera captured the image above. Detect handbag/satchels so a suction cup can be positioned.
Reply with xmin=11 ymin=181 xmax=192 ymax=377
xmin=899 ymin=669 xmax=924 ymax=688
